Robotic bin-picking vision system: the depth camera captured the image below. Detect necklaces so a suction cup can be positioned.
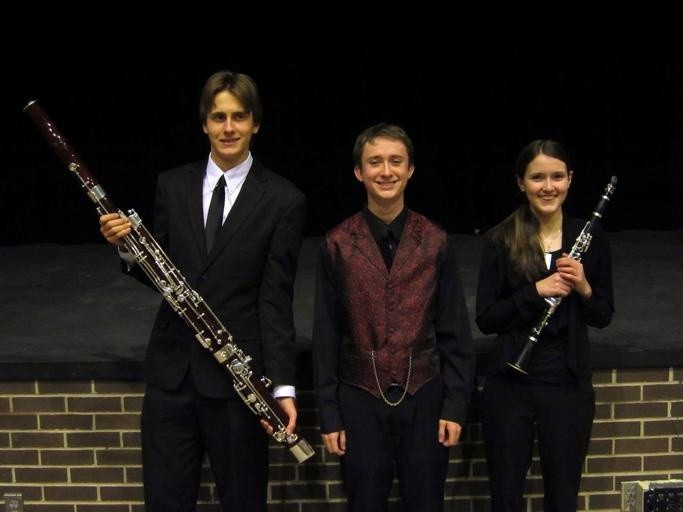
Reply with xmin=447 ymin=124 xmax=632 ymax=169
xmin=534 ymin=216 xmax=563 ymax=254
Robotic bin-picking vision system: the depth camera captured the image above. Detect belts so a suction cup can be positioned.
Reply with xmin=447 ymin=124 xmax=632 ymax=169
xmin=388 ymin=383 xmax=404 ymax=394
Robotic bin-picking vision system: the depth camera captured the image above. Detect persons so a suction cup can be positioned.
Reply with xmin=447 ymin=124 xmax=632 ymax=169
xmin=99 ymin=69 xmax=305 ymax=511
xmin=472 ymin=139 xmax=616 ymax=510
xmin=309 ymin=122 xmax=474 ymax=512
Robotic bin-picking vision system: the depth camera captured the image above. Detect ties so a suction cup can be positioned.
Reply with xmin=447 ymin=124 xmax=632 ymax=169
xmin=205 ymin=175 xmax=229 ymax=257
